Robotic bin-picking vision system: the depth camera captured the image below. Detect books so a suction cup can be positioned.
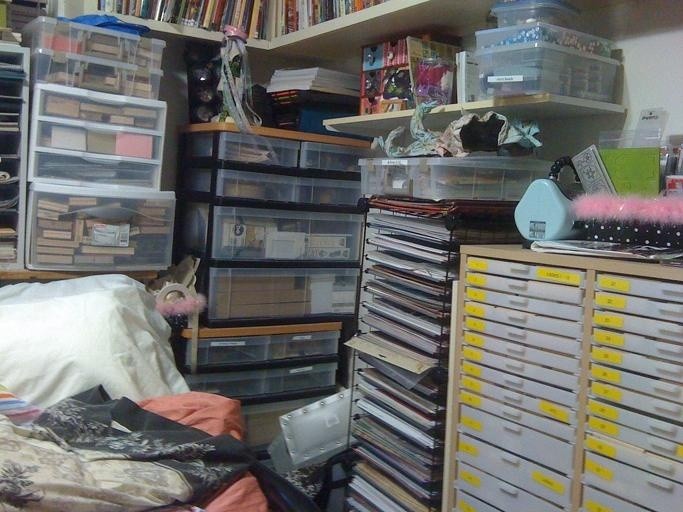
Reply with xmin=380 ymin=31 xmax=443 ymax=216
xmin=356 ymin=194 xmax=525 ymax=245
xmin=31 ymin=92 xmax=159 ymax=186
xmin=182 ymin=138 xmax=358 ymax=324
xmin=405 ymin=38 xmax=480 ymax=109
xmin=33 ymin=190 xmax=171 ymax=266
xmin=99 ymin=1 xmax=383 ymax=41
xmin=42 ymin=27 xmax=154 ymax=100
xmin=0 ymin=51 xmax=26 ymax=263
xmin=0 ymin=28 xmax=24 ymax=44
xmin=265 ymin=67 xmax=361 ymax=109
xmin=338 ymin=234 xmax=456 ymax=511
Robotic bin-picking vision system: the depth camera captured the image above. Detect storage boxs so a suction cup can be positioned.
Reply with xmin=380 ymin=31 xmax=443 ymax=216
xmin=357 ymin=157 xmax=559 ymax=202
xmin=180 ymin=322 xmax=344 ymax=405
xmin=475 ymin=0 xmax=621 ymax=102
xmin=27 ymin=84 xmax=168 ymax=191
xmin=25 ymin=184 xmax=176 ymax=274
xmin=240 ymin=398 xmax=333 ymax=478
xmin=173 ymin=123 xmax=375 ymax=328
xmin=18 ymin=16 xmax=167 ymax=100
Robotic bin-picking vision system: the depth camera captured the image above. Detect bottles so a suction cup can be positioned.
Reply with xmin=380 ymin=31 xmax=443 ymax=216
xmin=664 ymin=148 xmax=678 ymax=175
xmin=675 ymin=144 xmax=683 ymax=175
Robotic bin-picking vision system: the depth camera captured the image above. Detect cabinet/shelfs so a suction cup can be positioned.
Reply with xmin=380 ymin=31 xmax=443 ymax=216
xmin=63 ymin=0 xmax=627 ymax=136
xmin=447 ymin=244 xmax=683 ymax=511
xmin=342 ymin=199 xmax=533 ymax=511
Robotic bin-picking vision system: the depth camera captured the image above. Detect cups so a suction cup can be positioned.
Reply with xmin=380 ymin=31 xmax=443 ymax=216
xmin=665 ymin=175 xmax=683 ymax=195
